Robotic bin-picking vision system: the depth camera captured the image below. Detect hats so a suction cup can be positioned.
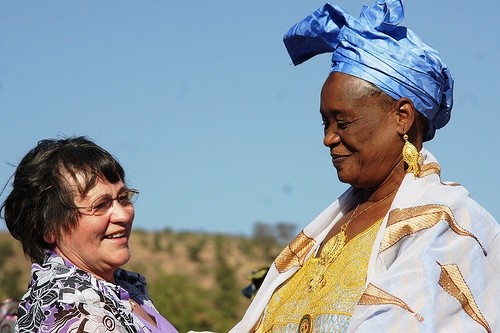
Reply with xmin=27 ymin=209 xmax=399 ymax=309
xmin=280 ymin=1 xmax=456 ymax=142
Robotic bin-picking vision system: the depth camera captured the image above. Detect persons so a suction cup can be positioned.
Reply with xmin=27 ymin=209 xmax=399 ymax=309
xmin=226 ymin=0 xmax=500 ymax=333
xmin=1 ymin=136 xmax=181 ymax=333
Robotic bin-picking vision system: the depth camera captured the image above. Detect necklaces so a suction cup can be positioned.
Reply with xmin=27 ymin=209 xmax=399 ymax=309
xmin=128 ymin=297 xmax=136 ymax=306
xmin=308 ymin=187 xmax=400 ymax=292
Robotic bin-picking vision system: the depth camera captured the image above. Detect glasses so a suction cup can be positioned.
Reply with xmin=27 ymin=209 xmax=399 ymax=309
xmin=65 ymin=187 xmax=139 ymax=217
